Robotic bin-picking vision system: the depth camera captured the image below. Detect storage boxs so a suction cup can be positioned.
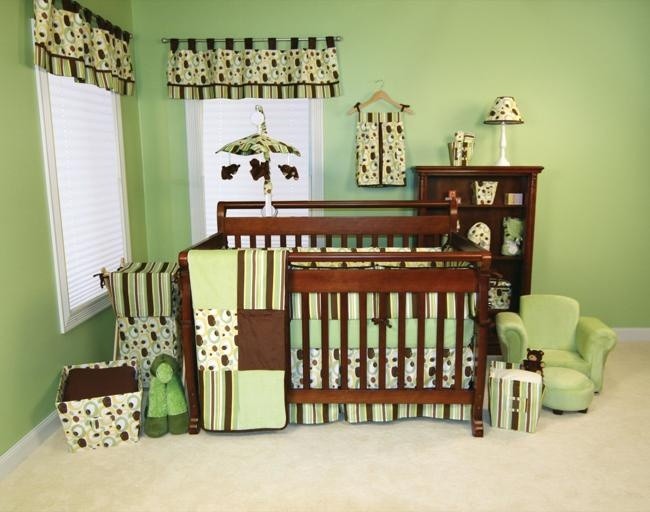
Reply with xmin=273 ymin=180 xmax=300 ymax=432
xmin=54 ymin=358 xmax=144 ymax=452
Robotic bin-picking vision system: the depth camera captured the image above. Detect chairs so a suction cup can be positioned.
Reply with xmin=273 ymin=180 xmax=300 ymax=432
xmin=496 ymin=295 xmax=616 ymax=413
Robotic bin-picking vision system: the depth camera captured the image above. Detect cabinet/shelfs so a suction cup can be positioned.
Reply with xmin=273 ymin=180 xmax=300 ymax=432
xmin=410 ymin=165 xmax=542 ymax=354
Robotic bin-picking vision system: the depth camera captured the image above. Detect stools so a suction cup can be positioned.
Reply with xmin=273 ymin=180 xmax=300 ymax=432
xmin=490 ymin=368 xmax=544 ymax=431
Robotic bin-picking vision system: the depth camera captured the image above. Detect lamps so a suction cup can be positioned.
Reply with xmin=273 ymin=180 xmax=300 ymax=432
xmin=484 ymin=97 xmax=525 ymax=166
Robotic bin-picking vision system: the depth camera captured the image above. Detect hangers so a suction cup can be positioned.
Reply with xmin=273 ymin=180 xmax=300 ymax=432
xmin=345 ymin=79 xmax=417 ymax=117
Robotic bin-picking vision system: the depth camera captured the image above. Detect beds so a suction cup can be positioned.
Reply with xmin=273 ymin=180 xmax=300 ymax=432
xmin=177 ymin=198 xmax=492 ymax=439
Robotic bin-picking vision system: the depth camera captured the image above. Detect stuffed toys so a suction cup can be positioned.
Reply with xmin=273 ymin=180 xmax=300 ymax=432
xmin=278 ymin=165 xmax=299 ymax=181
xmin=250 ymin=158 xmax=270 ymax=181
xmin=221 ymin=164 xmax=240 ymax=180
xmin=144 ymin=354 xmax=189 ymax=437
xmin=520 ymin=348 xmax=545 ymax=377
